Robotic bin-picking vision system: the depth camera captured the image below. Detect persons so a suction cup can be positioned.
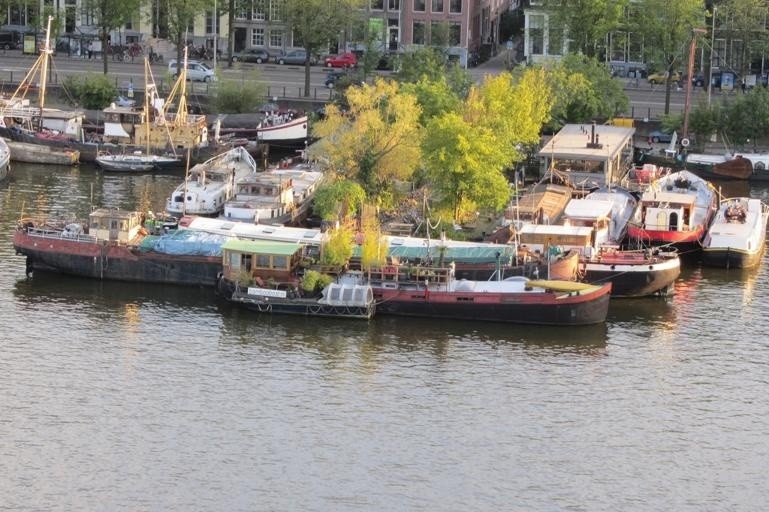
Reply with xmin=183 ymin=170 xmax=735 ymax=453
xmin=88 ymin=43 xmax=93 ymax=59
